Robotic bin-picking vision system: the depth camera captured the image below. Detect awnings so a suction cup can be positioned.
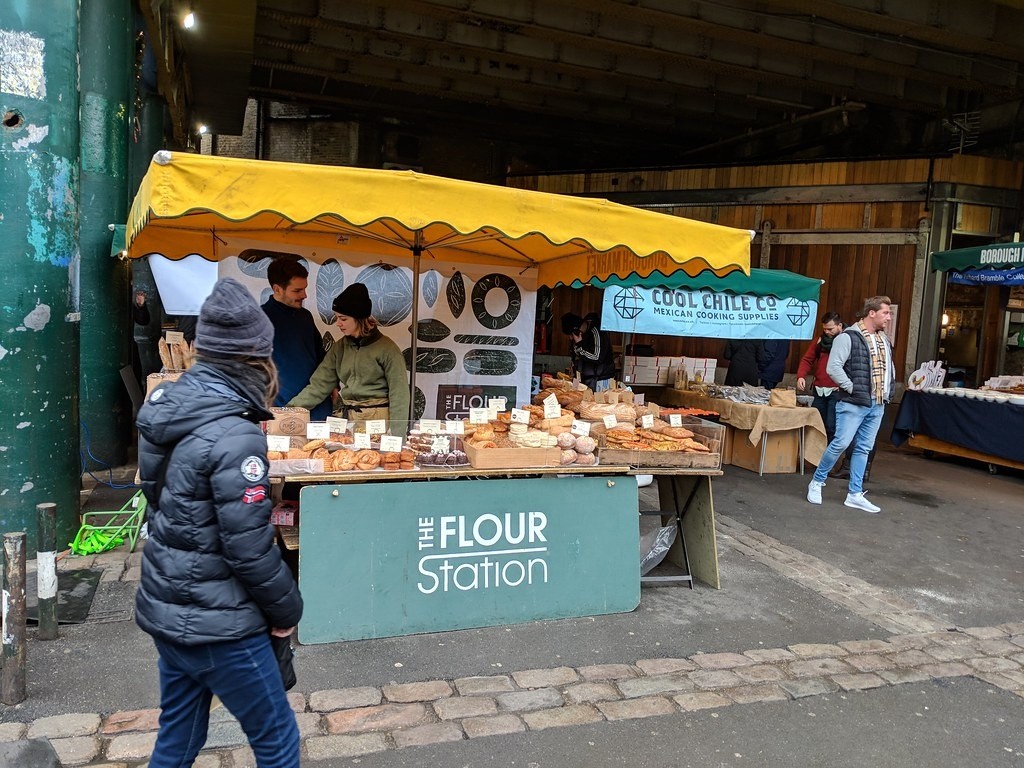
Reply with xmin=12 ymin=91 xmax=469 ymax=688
xmin=931 ymin=242 xmax=1024 ymax=273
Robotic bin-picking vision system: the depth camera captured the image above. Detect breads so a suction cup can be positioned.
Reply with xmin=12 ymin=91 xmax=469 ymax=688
xmin=266 ymin=371 xmax=652 ymax=472
xmin=158 ymin=336 xmax=196 ymax=373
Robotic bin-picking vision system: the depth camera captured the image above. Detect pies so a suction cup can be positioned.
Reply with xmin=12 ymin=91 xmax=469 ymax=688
xmin=603 ymin=427 xmax=710 ymax=453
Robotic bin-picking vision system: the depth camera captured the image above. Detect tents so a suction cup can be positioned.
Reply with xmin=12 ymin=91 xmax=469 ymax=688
xmin=556 ymin=269 xmax=825 ymax=303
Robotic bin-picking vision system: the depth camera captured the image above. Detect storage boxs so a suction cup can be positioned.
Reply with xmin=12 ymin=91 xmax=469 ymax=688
xmin=624 ymin=355 xmax=717 ymax=385
xmin=731 ymin=427 xmax=799 ymax=473
xmin=290 ymin=435 xmax=310 ymax=449
xmin=461 ymin=440 xmax=561 ymax=470
xmin=595 ymin=447 xmax=721 ymax=470
xmin=707 ymin=418 xmax=735 ymax=465
xmin=269 ymin=507 xmax=299 ymax=526
xmin=266 ymin=406 xmax=311 ymax=436
xmin=147 ymin=373 xmax=181 ymax=396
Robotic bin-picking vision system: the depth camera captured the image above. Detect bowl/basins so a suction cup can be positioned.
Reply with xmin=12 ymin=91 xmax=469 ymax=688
xmin=923 ymin=387 xmax=1024 ymax=405
xmin=796 ymin=395 xmax=814 ymax=407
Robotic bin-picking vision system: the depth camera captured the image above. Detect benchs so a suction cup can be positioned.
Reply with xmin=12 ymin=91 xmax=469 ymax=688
xmin=280 ymin=525 xmax=299 ymax=550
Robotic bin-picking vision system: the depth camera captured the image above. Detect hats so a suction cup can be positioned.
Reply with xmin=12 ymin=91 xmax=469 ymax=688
xmin=332 ymin=282 xmax=372 ymax=318
xmin=194 ymin=277 xmax=275 ymax=359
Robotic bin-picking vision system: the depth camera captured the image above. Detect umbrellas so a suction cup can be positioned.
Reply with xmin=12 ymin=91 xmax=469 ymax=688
xmin=124 ymin=151 xmax=755 ymax=433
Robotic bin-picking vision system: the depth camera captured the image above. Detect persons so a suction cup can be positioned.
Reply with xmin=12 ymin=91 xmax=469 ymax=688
xmin=134 ymin=278 xmax=303 ymax=768
xmin=285 ymin=284 xmax=410 ymax=444
xmin=807 ymin=297 xmax=895 ymax=512
xmin=260 ymin=260 xmax=342 ymax=421
xmin=561 ymin=313 xmax=614 ymax=391
xmin=723 ymin=339 xmax=791 ymax=389
xmin=797 ymin=314 xmax=877 ymax=481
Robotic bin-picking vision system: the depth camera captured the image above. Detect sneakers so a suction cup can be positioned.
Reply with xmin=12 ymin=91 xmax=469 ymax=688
xmin=843 ymin=491 xmax=881 ymax=513
xmin=807 ymin=479 xmax=826 ymax=504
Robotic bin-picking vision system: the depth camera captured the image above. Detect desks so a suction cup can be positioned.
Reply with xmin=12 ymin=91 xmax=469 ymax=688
xmin=626 ymin=468 xmax=725 ymax=590
xmin=663 ymin=388 xmax=817 ymax=477
xmin=625 ymin=383 xmax=674 ymax=387
xmin=893 ymin=389 xmax=1024 ymax=475
xmin=286 ymin=465 xmax=631 ymax=484
